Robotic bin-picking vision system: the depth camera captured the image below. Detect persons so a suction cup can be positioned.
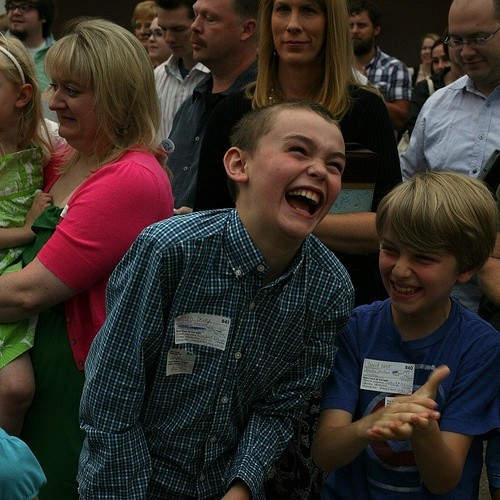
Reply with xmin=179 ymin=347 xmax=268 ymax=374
xmin=146 ymin=6 xmax=173 ymax=69
xmin=152 ymin=1 xmax=214 ymax=151
xmin=159 ymin=0 xmax=279 ymax=218
xmin=76 ymin=99 xmax=357 ymax=500
xmin=173 ymin=0 xmax=404 ymax=308
xmin=349 ymin=0 xmax=500 ymax=331
xmin=6 ymin=1 xmax=60 ymax=91
xmin=1 ymin=31 xmax=72 ymax=443
xmin=311 ymin=169 xmax=499 ymax=499
xmin=0 ymin=19 xmax=175 ymax=500
xmin=0 ymin=427 xmax=49 ymax=500
xmin=130 ymin=1 xmax=162 ymax=68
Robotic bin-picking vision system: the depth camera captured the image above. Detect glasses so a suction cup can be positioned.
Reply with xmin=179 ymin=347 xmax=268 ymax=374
xmin=444 ymin=28 xmax=498 ymax=48
xmin=4 ymin=1 xmax=34 ymax=13
